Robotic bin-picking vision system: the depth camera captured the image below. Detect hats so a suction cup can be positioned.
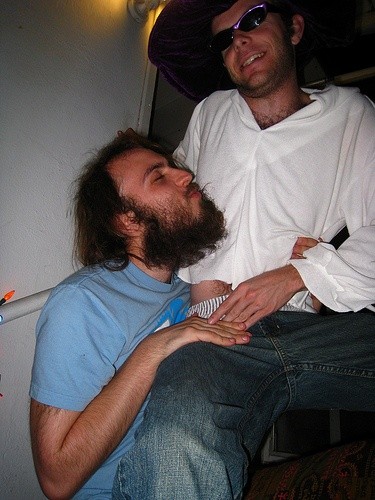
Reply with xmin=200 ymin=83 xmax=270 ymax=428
xmin=145 ymin=1 xmax=339 ymax=105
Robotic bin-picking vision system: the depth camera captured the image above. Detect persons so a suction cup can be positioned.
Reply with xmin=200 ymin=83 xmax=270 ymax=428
xmin=107 ymin=0 xmax=375 ymax=500
xmin=28 ymin=128 xmax=326 ymax=498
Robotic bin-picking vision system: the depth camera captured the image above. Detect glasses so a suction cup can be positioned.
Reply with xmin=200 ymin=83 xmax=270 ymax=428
xmin=209 ymin=2 xmax=279 ymax=53
